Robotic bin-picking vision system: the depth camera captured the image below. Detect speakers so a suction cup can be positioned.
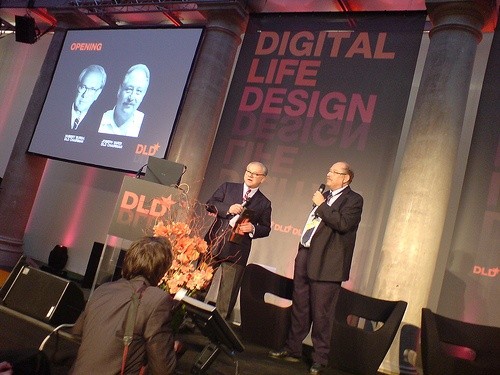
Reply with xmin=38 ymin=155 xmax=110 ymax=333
xmin=2 ymin=266 xmax=85 ymax=327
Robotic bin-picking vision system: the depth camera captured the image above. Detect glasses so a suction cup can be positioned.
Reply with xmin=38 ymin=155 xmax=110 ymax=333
xmin=245 ymin=168 xmax=267 ymax=179
xmin=76 ymin=83 xmax=103 ymax=94
xmin=327 ymin=168 xmax=348 ymax=177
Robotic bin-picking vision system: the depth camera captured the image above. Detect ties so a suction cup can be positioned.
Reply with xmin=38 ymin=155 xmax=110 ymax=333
xmin=300 ymin=191 xmax=335 ymax=244
xmin=239 ymin=188 xmax=252 ymax=211
xmin=71 ymin=113 xmax=81 ymax=132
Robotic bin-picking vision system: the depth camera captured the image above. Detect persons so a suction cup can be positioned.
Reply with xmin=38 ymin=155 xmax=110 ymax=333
xmin=177 ymin=161 xmax=272 ymax=338
xmin=97 ymin=64 xmax=151 ymax=138
xmin=50 ymin=65 xmax=107 ymax=133
xmin=267 ymin=160 xmax=364 ymax=375
xmin=66 ymin=234 xmax=178 ymax=375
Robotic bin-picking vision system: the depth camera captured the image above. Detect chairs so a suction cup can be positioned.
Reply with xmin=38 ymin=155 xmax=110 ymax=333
xmin=326 ymin=286 xmax=408 ymax=375
xmin=239 ymin=263 xmax=294 ymax=347
xmin=421 ymin=307 xmax=500 ymax=375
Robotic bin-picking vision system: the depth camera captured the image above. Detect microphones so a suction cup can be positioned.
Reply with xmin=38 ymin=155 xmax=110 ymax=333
xmin=313 ymin=184 xmax=326 ymax=207
xmin=136 ymin=158 xmax=165 ymax=178
xmin=230 ymin=198 xmax=251 ymax=216
xmin=175 ymin=166 xmax=187 ymax=188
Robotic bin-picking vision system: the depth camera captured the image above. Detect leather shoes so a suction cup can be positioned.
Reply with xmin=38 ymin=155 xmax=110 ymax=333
xmin=268 ymin=346 xmax=301 ymax=363
xmin=309 ymin=361 xmax=328 ymax=374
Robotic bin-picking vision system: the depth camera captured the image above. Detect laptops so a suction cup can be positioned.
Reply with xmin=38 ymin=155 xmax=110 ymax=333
xmin=144 ymin=156 xmax=184 ymax=187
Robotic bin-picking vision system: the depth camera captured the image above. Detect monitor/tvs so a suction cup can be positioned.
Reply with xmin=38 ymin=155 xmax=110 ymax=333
xmin=183 ymin=295 xmax=244 ymax=353
